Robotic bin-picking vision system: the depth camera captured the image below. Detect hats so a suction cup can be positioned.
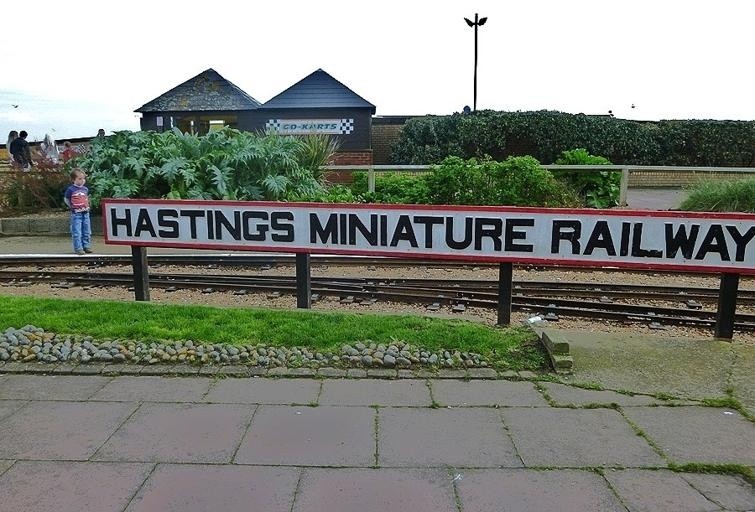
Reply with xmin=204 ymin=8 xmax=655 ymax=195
xmin=20 ymin=131 xmax=28 ymax=135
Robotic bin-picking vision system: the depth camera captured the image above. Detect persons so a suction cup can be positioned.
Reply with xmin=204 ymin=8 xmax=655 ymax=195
xmin=62 ymin=141 xmax=78 ymax=164
xmin=63 ymin=168 xmax=94 ymax=255
xmin=5 ymin=130 xmax=22 ymax=167
xmin=37 ymin=133 xmax=60 ymax=168
xmin=8 ymin=130 xmax=36 ymax=168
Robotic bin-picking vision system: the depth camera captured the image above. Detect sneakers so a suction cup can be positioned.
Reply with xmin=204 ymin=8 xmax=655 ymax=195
xmin=74 ymin=250 xmax=85 ymax=255
xmin=85 ymin=248 xmax=93 ymax=253
